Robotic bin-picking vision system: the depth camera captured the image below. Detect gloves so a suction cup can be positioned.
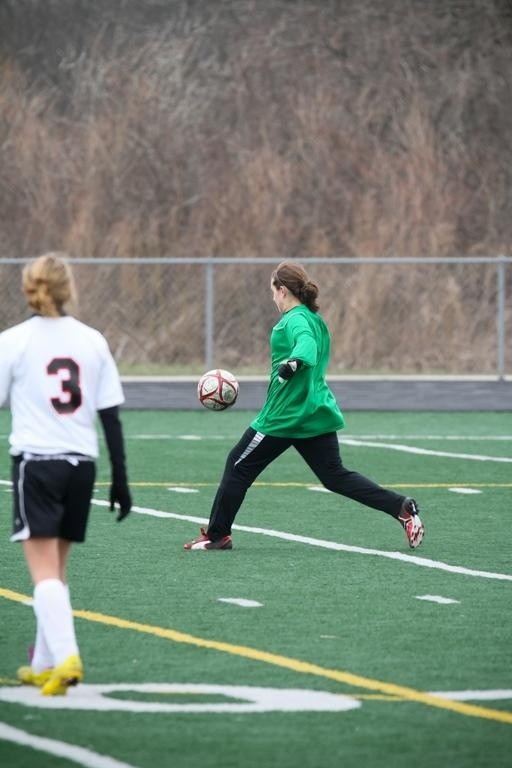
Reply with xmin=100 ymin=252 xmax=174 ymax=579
xmin=109 ymin=477 xmax=133 ymax=522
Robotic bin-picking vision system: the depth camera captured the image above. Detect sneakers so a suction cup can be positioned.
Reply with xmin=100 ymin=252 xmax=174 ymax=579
xmin=183 ymin=532 xmax=234 ymax=551
xmin=17 ymin=665 xmax=55 ymax=688
xmin=398 ymin=496 xmax=425 ymax=550
xmin=41 ymin=655 xmax=82 ymax=697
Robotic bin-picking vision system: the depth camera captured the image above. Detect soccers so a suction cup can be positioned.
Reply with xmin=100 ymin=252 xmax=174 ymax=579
xmin=197 ymin=369 xmax=239 ymax=410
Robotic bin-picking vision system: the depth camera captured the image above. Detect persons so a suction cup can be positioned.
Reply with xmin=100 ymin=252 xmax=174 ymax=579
xmin=184 ymin=259 xmax=425 ymax=552
xmin=0 ymin=252 xmax=133 ymax=696
